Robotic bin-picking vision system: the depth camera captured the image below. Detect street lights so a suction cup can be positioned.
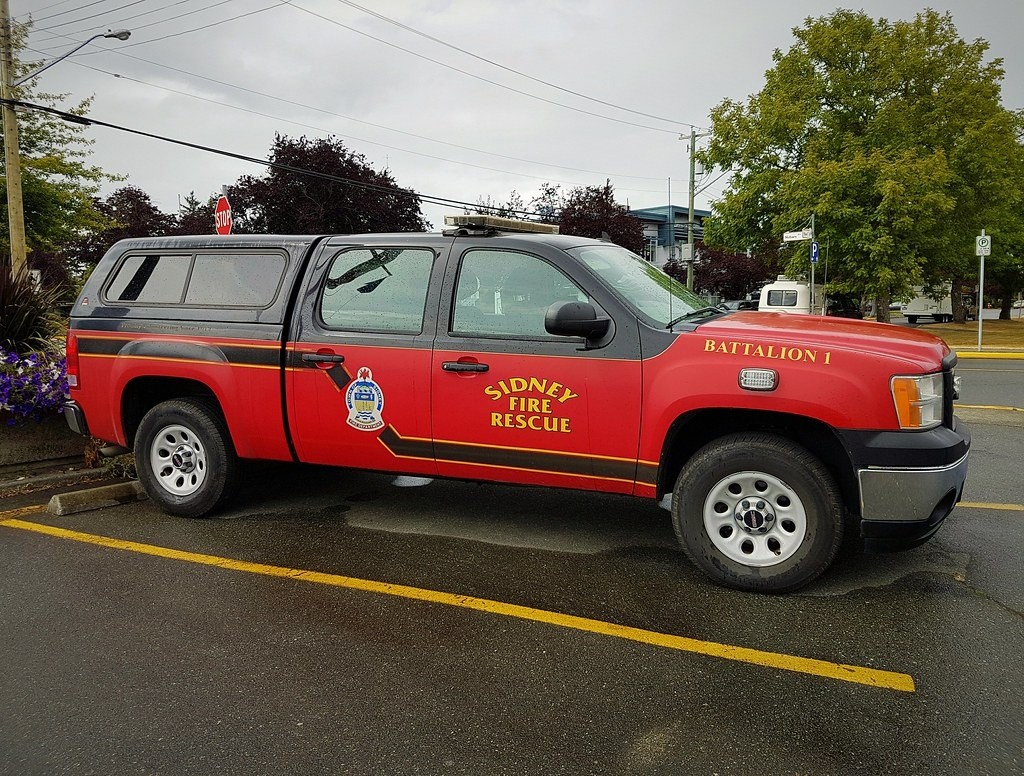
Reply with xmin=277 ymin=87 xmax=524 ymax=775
xmin=1 ymin=29 xmax=133 ymax=290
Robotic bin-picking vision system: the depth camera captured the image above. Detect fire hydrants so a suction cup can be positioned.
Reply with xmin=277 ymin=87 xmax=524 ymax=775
xmin=987 ymin=303 xmax=991 ymax=309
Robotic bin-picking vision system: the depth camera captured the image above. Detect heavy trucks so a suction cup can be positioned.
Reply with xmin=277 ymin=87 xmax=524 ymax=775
xmin=902 ymin=278 xmax=975 ymax=323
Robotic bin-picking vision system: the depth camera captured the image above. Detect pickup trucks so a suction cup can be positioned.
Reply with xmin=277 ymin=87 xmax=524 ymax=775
xmin=715 ymin=300 xmax=759 ymax=311
xmin=64 ymin=226 xmax=973 ymax=594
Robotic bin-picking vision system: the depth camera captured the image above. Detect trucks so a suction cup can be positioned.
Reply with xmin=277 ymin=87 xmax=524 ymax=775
xmin=758 ymin=273 xmax=834 ymax=316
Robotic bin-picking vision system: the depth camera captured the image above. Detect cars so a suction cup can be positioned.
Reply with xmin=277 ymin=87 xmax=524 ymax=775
xmin=1014 ymin=300 xmax=1024 ymax=309
xmin=888 ymin=300 xmax=902 ymax=311
xmin=825 ymin=294 xmax=863 ymax=320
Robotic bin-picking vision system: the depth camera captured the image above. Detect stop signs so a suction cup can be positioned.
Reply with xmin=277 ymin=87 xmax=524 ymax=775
xmin=214 ymin=196 xmax=234 ymax=236
